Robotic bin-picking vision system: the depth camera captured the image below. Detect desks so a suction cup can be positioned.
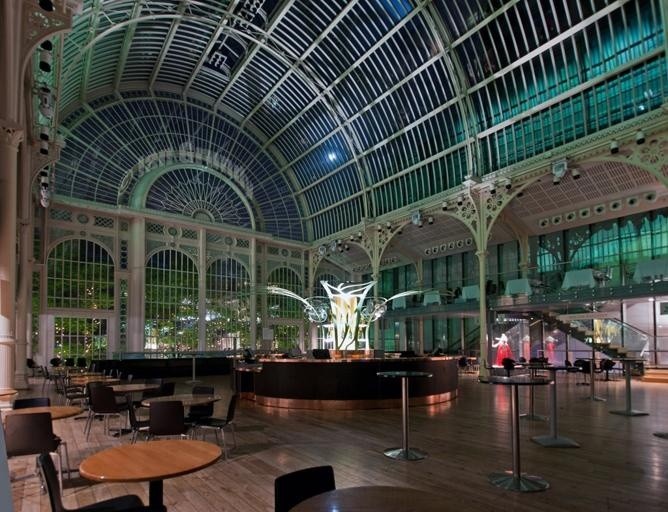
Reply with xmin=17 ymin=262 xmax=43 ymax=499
xmin=377 ymin=371 xmax=433 ymax=460
xmin=79 ymin=438 xmax=223 ymax=512
xmin=386 ymin=258 xmax=667 ymax=311
xmin=289 ymin=486 xmax=458 ymax=511
xmin=477 ymin=356 xmax=647 ymax=492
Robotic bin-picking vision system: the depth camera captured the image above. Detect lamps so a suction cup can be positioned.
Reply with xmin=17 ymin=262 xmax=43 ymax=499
xmin=29 ymin=0 xmax=53 ymax=191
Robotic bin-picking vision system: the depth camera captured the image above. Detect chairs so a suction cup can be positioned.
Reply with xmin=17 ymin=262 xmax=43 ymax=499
xmin=34 ymin=454 xmax=143 ymax=512
xmin=272 ymin=465 xmax=335 ymax=511
xmin=0 ymin=368 xmax=237 ymax=492
